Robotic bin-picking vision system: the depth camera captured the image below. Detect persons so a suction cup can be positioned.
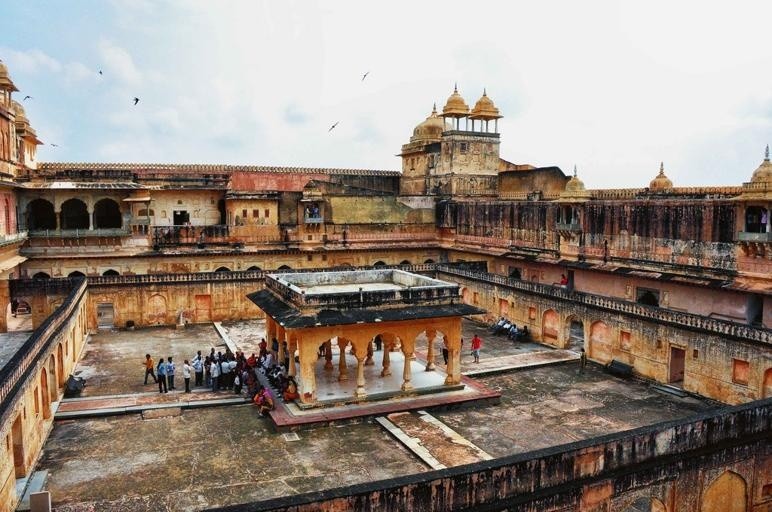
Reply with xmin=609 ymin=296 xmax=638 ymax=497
xmin=492 ymin=317 xmax=528 ymax=342
xmin=305 ymin=205 xmax=320 ymax=218
xmin=560 ymin=274 xmax=568 ymax=289
xmin=374 ymin=335 xmax=382 ymax=350
xmin=461 ymin=338 xmax=463 ymax=350
xmin=471 ymin=334 xmax=481 ymax=363
xmin=747 ymin=208 xmax=767 ymax=233
xmin=144 ymin=336 xmax=300 ymax=419
xmin=440 ymin=337 xmax=448 ymax=365
xmin=319 ymin=343 xmax=326 ymax=356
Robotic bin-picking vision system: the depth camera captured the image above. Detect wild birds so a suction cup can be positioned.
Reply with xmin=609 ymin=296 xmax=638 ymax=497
xmin=360 ymin=70 xmax=371 ymax=81
xmin=50 ymin=144 xmax=60 ymax=148
xmin=98 ymin=70 xmax=103 ymax=75
xmin=23 ymin=95 xmax=34 ymax=101
xmin=133 ymin=97 xmax=139 ymax=105
xmin=328 ymin=120 xmax=340 ymax=131
